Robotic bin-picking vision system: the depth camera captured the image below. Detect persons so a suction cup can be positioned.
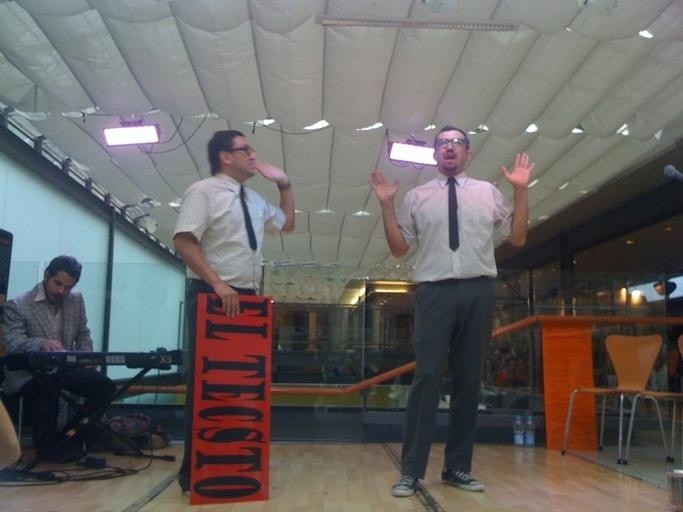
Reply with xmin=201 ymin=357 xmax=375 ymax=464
xmin=0 ymin=253 xmax=119 ymax=464
xmin=170 ymin=127 xmax=296 ymax=498
xmin=367 ymin=124 xmax=536 ymax=498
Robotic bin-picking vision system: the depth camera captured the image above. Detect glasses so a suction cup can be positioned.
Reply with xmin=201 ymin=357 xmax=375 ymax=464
xmin=230 ymin=145 xmax=254 ymax=155
xmin=436 ymin=139 xmax=465 ymax=147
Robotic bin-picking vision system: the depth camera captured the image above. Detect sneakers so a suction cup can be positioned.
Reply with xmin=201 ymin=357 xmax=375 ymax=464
xmin=392 ymin=475 xmax=417 ymax=496
xmin=441 ymin=469 xmax=484 ymax=491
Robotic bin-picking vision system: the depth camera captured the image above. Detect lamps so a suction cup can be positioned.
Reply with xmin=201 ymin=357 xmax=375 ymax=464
xmin=103 ymin=120 xmax=160 ymax=147
xmin=387 ymin=140 xmax=437 ymax=168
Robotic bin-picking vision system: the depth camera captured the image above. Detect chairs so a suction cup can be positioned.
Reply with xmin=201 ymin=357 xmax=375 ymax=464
xmin=561 ymin=334 xmax=683 ymax=464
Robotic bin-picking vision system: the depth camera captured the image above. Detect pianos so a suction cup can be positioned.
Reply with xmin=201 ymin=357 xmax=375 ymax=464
xmin=3 ymin=352 xmax=183 ymax=369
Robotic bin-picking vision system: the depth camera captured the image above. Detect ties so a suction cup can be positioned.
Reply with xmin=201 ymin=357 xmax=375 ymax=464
xmin=240 ymin=185 xmax=257 ymax=251
xmin=446 ymin=176 xmax=459 ymax=252
